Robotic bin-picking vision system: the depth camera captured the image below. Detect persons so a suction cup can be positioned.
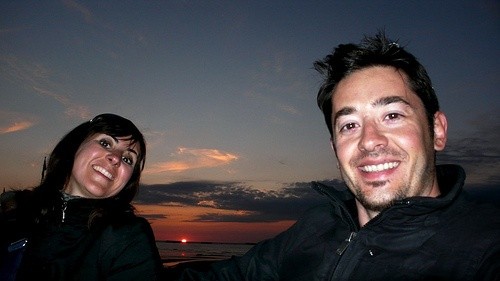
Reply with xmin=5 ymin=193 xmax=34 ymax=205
xmin=0 ymin=114 xmax=163 ymax=281
xmin=162 ymin=32 xmax=500 ymax=280
